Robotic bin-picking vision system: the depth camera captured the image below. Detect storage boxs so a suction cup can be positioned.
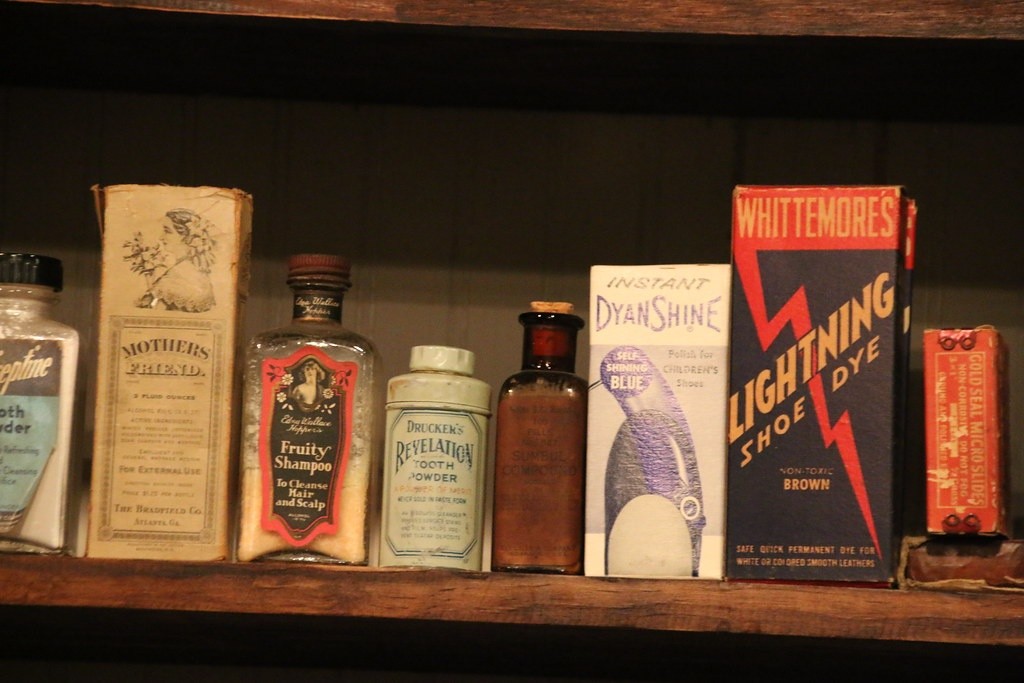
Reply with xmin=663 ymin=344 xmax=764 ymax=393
xmin=723 ymin=186 xmax=917 ymax=587
xmin=583 ymin=262 xmax=731 ymax=583
xmin=85 ymin=182 xmax=255 ymax=562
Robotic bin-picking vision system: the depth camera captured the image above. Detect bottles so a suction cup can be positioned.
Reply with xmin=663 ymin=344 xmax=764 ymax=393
xmin=490 ymin=301 xmax=592 ymax=575
xmin=380 ymin=344 xmax=492 ymax=571
xmin=235 ymin=252 xmax=383 ymax=568
xmin=0 ymin=251 xmax=80 ymax=558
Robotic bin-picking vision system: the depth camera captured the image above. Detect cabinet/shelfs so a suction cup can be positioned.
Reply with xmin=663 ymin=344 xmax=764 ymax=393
xmin=0 ymin=0 xmax=1024 ymax=683
